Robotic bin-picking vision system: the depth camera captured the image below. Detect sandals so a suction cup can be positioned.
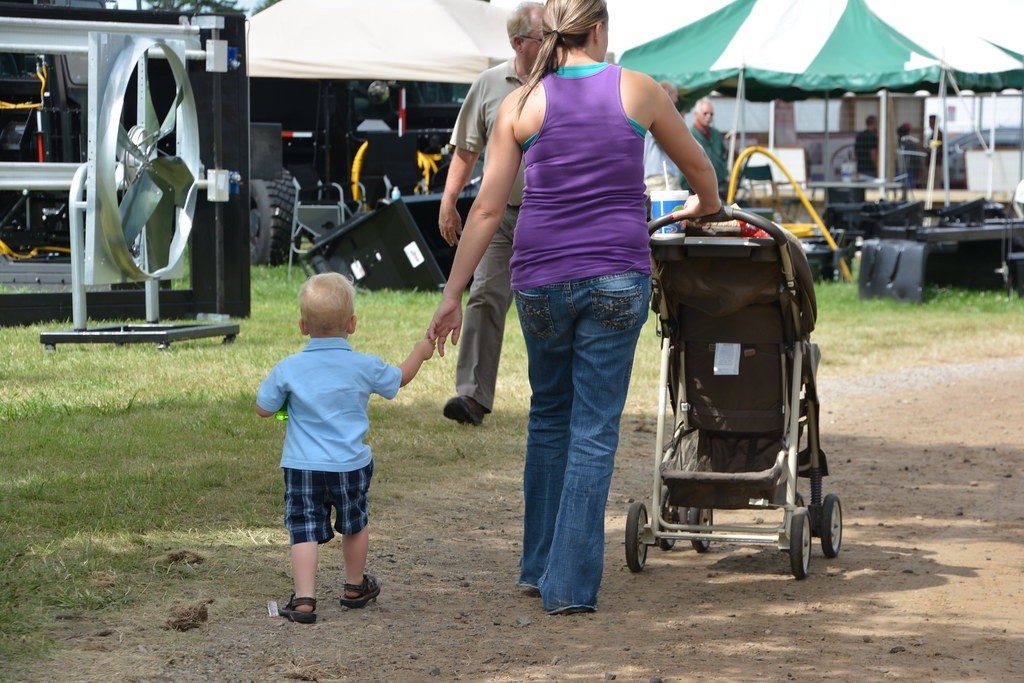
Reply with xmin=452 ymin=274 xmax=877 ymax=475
xmin=340 ymin=573 xmax=379 ymax=608
xmin=280 ymin=593 xmax=317 ymax=623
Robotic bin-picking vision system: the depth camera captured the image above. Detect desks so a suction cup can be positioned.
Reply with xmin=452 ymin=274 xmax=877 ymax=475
xmin=736 ymin=188 xmax=1009 ymax=223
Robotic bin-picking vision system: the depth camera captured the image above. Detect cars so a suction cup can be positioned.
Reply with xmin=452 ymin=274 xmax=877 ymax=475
xmin=939 ymin=122 xmax=1023 ymax=184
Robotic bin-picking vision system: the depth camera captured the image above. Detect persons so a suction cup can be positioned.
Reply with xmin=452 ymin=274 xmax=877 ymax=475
xmin=854 ymin=115 xmax=943 ymax=182
xmin=254 ymin=273 xmax=436 ymax=623
xmin=438 ymin=0 xmax=736 ymax=617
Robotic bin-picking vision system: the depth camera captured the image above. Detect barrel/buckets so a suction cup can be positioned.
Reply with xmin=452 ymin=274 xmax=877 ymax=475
xmin=649 ymin=189 xmax=690 ymax=233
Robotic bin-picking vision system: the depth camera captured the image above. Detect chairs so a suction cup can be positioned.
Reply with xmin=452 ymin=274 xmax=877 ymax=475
xmin=745 ymin=163 xmax=802 ymax=223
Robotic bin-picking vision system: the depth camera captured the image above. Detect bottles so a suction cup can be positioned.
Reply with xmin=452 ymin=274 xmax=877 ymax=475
xmin=391 ymin=186 xmax=400 ymax=202
xmin=274 ymin=397 xmax=289 ymax=421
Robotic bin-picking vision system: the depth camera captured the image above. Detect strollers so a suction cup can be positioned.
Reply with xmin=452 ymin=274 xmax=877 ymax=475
xmin=623 ymin=205 xmax=844 ymax=580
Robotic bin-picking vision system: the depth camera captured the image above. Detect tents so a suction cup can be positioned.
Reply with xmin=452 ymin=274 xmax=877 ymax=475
xmin=614 ymin=0 xmax=1024 ymax=222
xmin=246 ymin=0 xmax=517 ymax=204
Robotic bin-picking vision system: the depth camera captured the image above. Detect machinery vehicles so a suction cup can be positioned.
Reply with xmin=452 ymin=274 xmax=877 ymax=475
xmin=0 ymin=1 xmax=483 ymax=293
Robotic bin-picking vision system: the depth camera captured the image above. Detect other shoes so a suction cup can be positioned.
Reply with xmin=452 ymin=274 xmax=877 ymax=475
xmin=443 ymin=394 xmax=483 ymax=426
